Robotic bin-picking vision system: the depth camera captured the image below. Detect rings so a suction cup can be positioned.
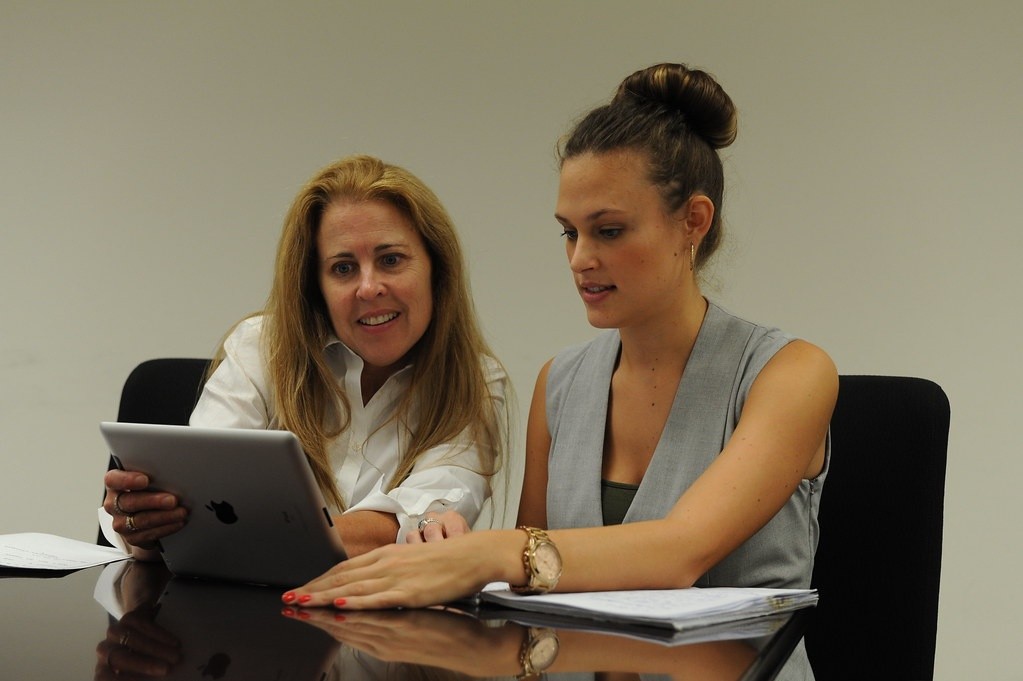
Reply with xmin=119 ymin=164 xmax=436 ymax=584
xmin=125 ymin=513 xmax=138 ymax=532
xmin=104 ymin=648 xmax=114 ymax=669
xmin=113 ymin=493 xmax=123 ymax=514
xmin=418 ymin=518 xmax=441 ymax=532
xmin=119 ymin=630 xmax=131 ymax=645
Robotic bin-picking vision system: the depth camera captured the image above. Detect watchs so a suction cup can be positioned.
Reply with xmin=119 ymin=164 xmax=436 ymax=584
xmin=518 ymin=626 xmax=560 ymax=681
xmin=510 ymin=526 xmax=564 ymax=595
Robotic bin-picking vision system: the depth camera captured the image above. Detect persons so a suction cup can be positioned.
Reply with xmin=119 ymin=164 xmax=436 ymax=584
xmin=102 ymin=154 xmax=508 ymax=561
xmin=92 ymin=560 xmax=459 ymax=681
xmin=280 ymin=63 xmax=840 ymax=681
xmin=282 ymin=605 xmax=760 ymax=681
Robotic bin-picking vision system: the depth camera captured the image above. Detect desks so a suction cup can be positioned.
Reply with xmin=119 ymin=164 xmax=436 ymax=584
xmin=0 ymin=557 xmax=820 ymax=681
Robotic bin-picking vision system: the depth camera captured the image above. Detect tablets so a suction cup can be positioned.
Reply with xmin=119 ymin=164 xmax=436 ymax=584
xmin=126 ymin=575 xmax=343 ymax=681
xmin=102 ymin=422 xmax=349 ymax=587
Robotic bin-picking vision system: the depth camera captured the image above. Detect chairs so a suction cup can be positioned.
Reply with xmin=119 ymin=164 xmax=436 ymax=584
xmin=804 ymin=376 xmax=952 ymax=681
xmin=97 ymin=359 xmax=219 ymax=545
